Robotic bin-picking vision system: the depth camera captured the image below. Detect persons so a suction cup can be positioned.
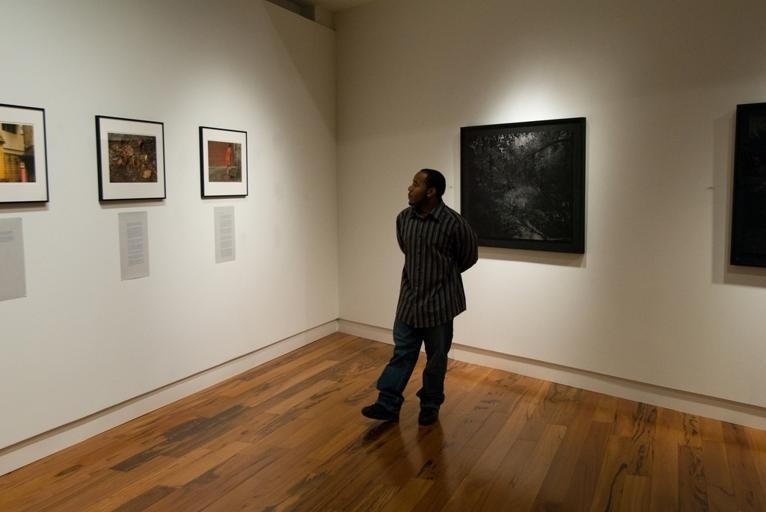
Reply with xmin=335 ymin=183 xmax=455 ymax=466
xmin=225 ymin=143 xmax=234 ymax=179
xmin=361 ymin=168 xmax=480 ymax=426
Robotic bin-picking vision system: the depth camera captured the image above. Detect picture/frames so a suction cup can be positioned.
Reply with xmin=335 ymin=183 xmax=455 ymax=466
xmin=730 ymin=103 xmax=766 ymax=267
xmin=460 ymin=117 xmax=584 ymax=253
xmin=0 ymin=104 xmax=49 ymax=204
xmin=96 ymin=116 xmax=166 ymax=201
xmin=201 ymin=126 xmax=248 ymax=197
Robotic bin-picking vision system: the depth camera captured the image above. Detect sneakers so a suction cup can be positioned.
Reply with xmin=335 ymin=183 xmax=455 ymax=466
xmin=361 ymin=403 xmax=398 ymax=420
xmin=419 ymin=403 xmax=439 ymax=423
